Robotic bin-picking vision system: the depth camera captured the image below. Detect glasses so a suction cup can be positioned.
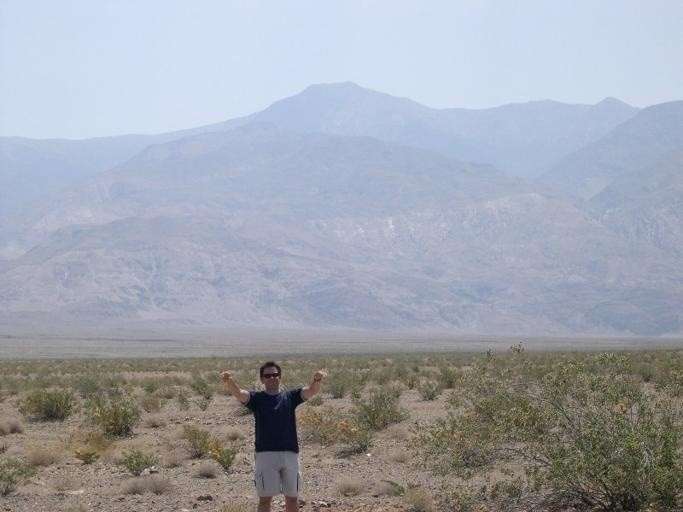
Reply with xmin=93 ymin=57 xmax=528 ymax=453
xmin=261 ymin=373 xmax=280 ymax=379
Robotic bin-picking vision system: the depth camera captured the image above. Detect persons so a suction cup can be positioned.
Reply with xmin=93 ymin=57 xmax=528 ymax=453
xmin=223 ymin=362 xmax=327 ymax=512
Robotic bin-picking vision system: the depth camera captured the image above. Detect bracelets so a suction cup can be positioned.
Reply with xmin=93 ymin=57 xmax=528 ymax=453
xmin=314 ymin=379 xmax=322 ymax=382
